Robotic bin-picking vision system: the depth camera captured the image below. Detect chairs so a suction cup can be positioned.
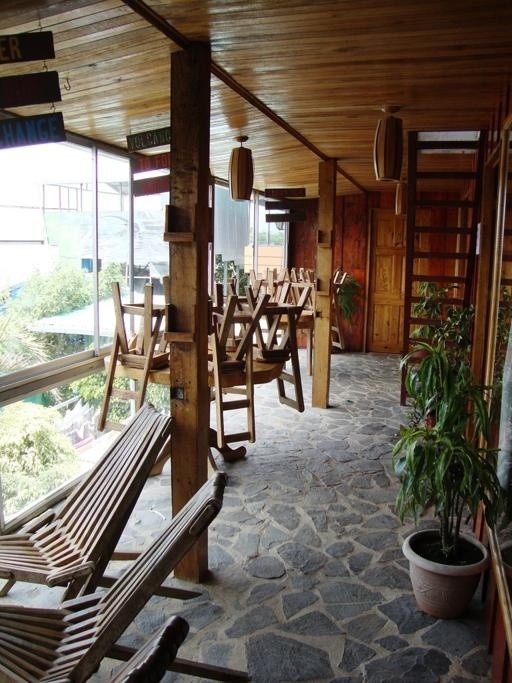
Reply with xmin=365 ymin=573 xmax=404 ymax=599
xmin=0 ymin=471 xmax=251 ymax=683
xmin=228 ymin=282 xmax=309 ymax=421
xmin=0 ymin=402 xmax=203 ymax=601
xmin=326 ymin=270 xmax=346 ymax=350
xmin=208 ymin=284 xmax=272 ymax=450
xmin=103 ymin=616 xmax=195 ymax=683
xmin=98 ymin=278 xmax=169 ymax=432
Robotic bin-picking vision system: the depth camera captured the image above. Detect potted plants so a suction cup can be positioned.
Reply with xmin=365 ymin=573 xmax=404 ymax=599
xmin=390 ymin=338 xmax=490 ymax=619
xmin=331 ymin=268 xmax=359 ymax=354
xmin=404 ymin=279 xmax=476 ymax=430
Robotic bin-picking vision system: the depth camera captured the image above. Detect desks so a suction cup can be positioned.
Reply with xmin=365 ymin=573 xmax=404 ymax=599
xmin=101 ymin=343 xmax=284 ymax=476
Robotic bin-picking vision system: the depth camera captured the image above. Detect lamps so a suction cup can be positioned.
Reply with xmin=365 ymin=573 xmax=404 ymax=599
xmin=374 ymin=103 xmax=404 ymax=182
xmin=228 ymin=135 xmax=254 ymax=201
